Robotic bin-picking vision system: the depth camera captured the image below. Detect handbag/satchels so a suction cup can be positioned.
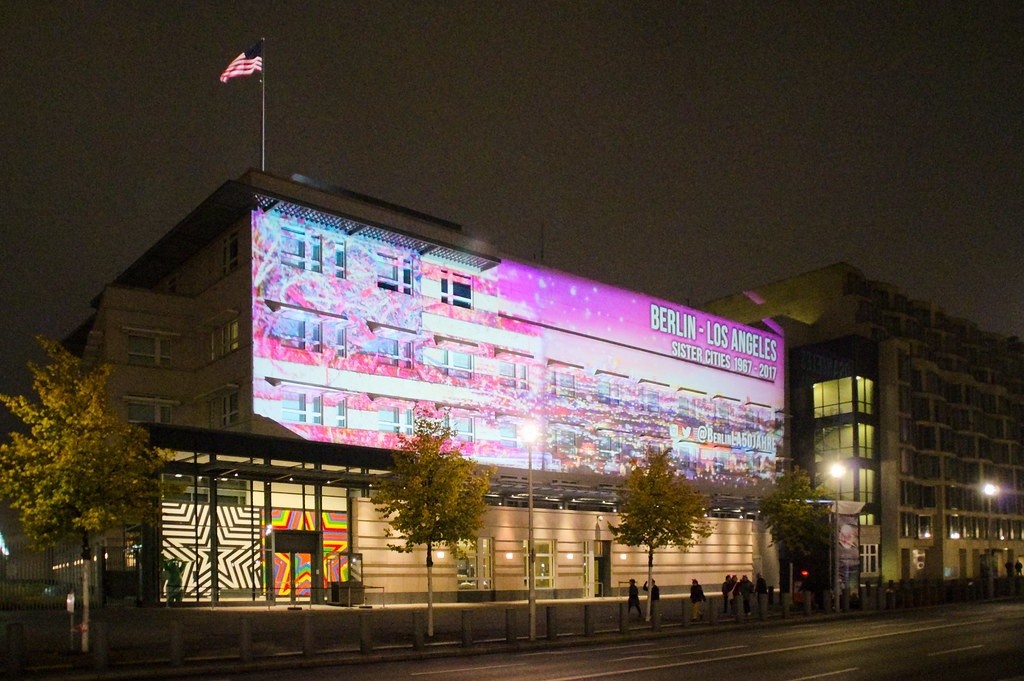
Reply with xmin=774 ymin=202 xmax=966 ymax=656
xmin=728 ymin=591 xmax=734 ymax=600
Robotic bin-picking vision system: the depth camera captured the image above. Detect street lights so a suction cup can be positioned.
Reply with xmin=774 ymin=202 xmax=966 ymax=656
xmin=986 ymin=483 xmax=995 ymax=597
xmin=516 ymin=422 xmax=541 ymax=641
xmin=830 ymin=462 xmax=846 ymax=612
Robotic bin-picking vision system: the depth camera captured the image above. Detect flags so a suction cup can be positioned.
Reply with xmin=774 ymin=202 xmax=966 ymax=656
xmin=220 ymin=41 xmax=263 ymax=82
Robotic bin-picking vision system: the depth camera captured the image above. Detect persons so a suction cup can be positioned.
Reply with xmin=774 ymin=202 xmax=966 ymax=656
xmin=722 ymin=573 xmax=767 ymax=615
xmin=690 ymin=579 xmax=705 ymax=621
xmin=1005 ymin=559 xmax=1023 ymax=577
xmin=643 ymin=579 xmax=659 ymax=617
xmin=163 ymin=557 xmax=186 ymax=606
xmin=628 ymin=580 xmax=641 ymax=618
